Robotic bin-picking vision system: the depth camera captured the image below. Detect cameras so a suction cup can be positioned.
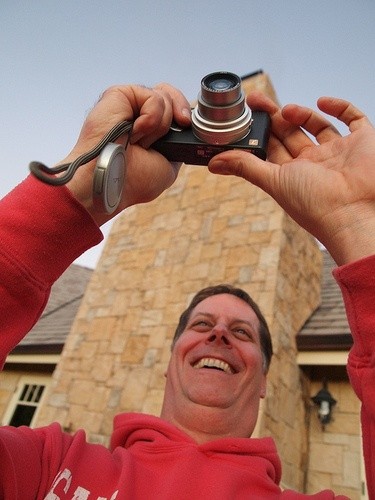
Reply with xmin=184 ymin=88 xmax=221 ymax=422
xmin=151 ymin=72 xmax=271 ymax=165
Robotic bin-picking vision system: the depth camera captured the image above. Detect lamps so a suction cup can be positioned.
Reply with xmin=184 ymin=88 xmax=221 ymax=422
xmin=312 ymin=385 xmax=337 ymax=429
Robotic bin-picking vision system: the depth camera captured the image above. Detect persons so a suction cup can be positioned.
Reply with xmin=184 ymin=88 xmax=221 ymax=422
xmin=0 ymin=83 xmax=375 ymax=500
